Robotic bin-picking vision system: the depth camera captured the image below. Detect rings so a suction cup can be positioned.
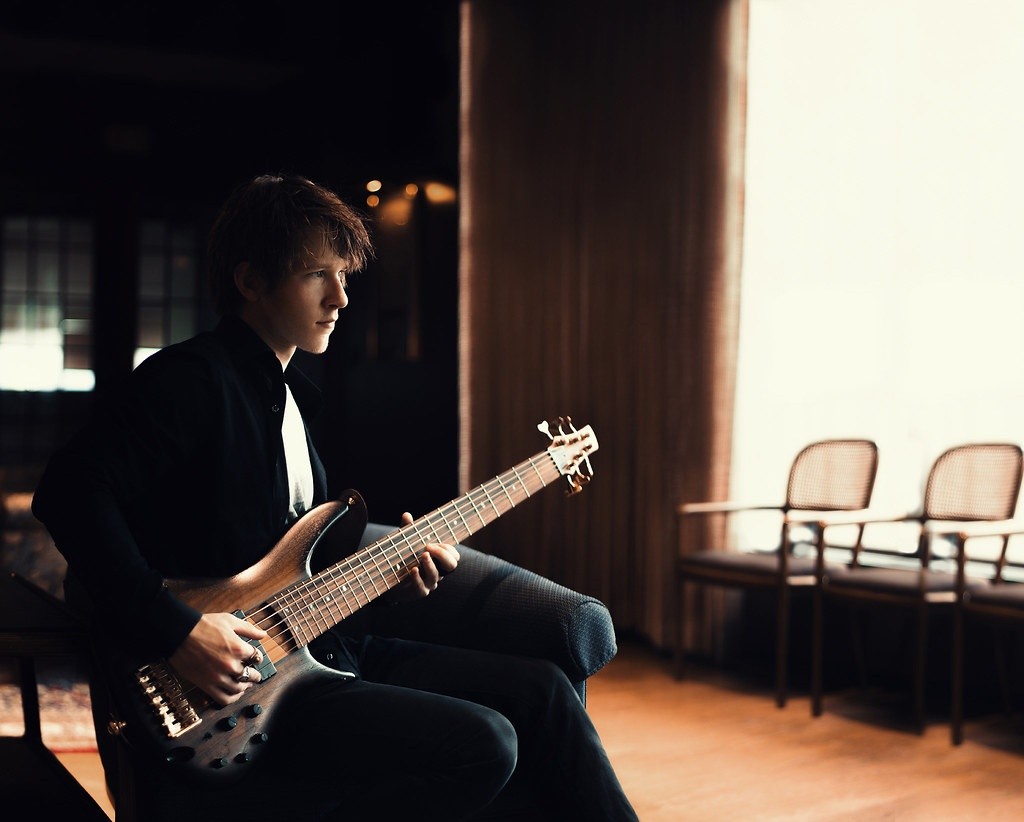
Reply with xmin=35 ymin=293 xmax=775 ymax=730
xmin=237 ymin=666 xmax=251 ymax=682
xmin=242 ymin=646 xmax=260 ymax=663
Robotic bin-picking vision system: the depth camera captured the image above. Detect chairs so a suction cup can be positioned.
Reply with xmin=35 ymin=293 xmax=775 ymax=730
xmin=805 ymin=443 xmax=1024 ymax=736
xmin=670 ymin=439 xmax=880 ymax=710
xmin=945 ymin=523 xmax=1024 ymax=747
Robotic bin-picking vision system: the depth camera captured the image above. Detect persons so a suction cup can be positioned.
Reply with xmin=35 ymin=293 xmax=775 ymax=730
xmin=31 ymin=173 xmax=643 ymax=822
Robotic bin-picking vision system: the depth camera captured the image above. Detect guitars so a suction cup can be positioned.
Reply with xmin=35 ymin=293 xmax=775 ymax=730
xmin=89 ymin=416 xmax=600 ymax=787
